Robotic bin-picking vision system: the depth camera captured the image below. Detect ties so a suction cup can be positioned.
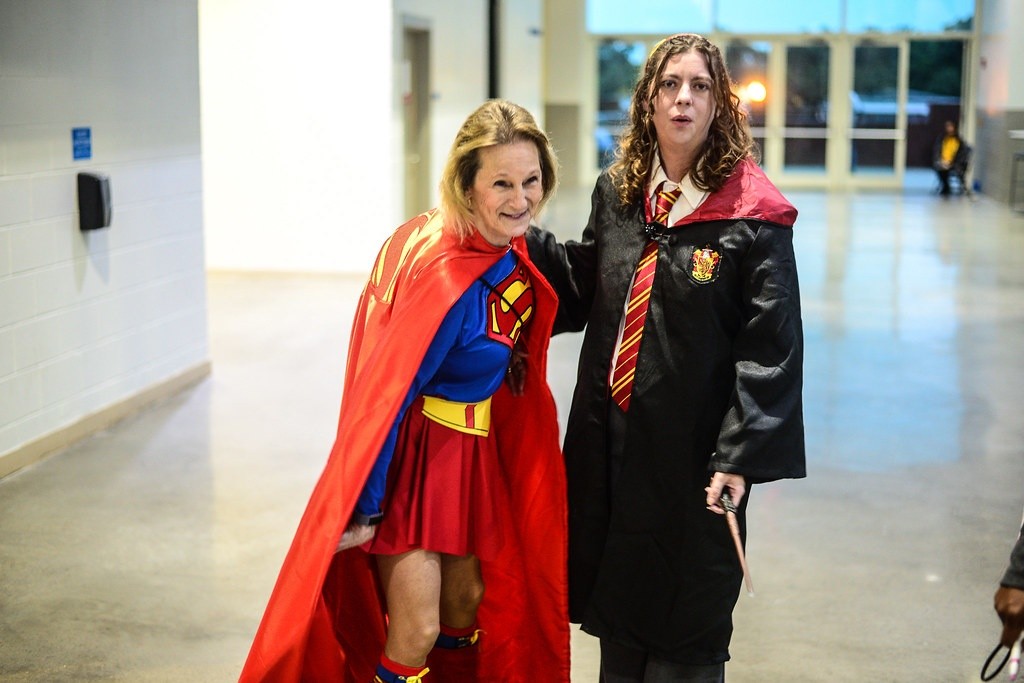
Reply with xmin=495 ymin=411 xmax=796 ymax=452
xmin=612 ymin=182 xmax=682 ymax=412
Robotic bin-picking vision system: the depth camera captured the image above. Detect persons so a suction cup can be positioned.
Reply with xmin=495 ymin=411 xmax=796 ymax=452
xmin=995 ymin=519 xmax=1024 ymax=650
xmin=522 ymin=33 xmax=807 ymax=683
xmin=932 ymin=119 xmax=967 ymax=196
xmin=235 ymin=98 xmax=559 ymax=683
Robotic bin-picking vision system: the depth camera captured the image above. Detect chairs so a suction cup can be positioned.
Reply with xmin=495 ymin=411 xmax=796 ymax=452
xmin=934 ymin=146 xmax=976 ymax=198
